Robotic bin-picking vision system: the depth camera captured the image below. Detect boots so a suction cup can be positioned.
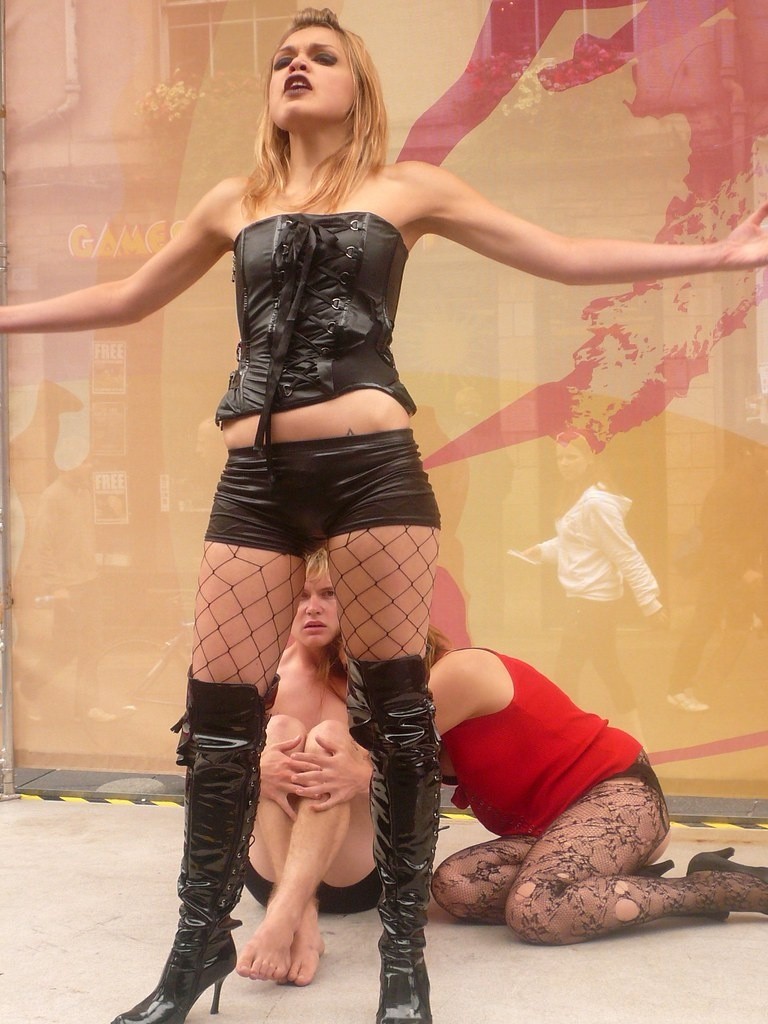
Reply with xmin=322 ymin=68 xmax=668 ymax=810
xmin=343 ymin=654 xmax=442 ymax=1024
xmin=113 ymin=673 xmax=282 ymax=1024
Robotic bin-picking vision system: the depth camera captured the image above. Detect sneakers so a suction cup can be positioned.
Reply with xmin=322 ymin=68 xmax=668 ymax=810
xmin=69 ymin=701 xmax=117 ymax=726
xmin=14 ymin=683 xmax=43 ymax=726
xmin=666 ymin=692 xmax=712 ymax=716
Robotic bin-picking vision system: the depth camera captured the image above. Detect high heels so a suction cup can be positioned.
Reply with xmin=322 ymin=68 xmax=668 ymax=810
xmin=633 ymin=859 xmax=731 ymax=922
xmin=687 ymin=846 xmax=768 ymax=886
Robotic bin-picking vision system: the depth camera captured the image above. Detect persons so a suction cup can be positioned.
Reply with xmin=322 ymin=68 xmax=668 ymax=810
xmin=425 ymin=624 xmax=768 ymax=946
xmin=15 ymin=437 xmax=117 ymax=726
xmin=521 ymin=428 xmax=670 ymax=759
xmin=667 ymin=423 xmax=768 ymax=714
xmin=0 ymin=8 xmax=768 ymax=1024
xmin=231 ymin=538 xmax=455 ymax=986
